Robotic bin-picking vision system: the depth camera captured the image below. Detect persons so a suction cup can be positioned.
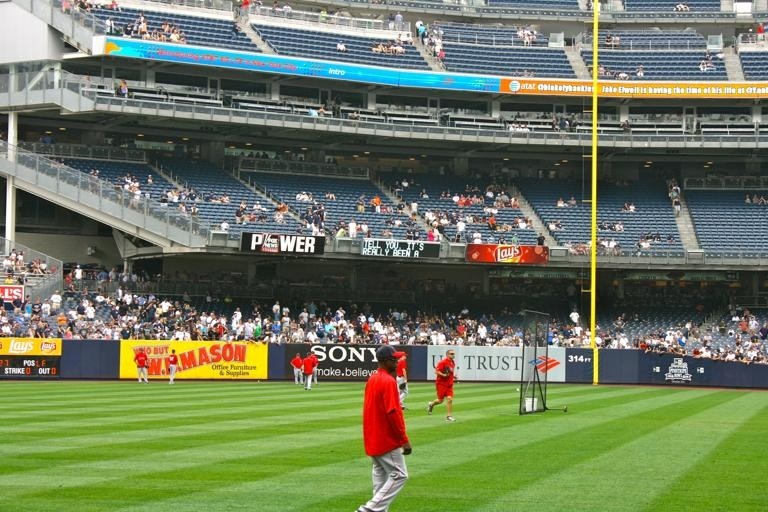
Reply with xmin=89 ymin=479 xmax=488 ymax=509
xmin=620 ymin=120 xmax=631 ymax=141
xmin=396 ymin=352 xmax=409 ymax=411
xmin=240 ymin=0 xmax=293 ymax=18
xmin=317 ymin=9 xmax=340 ymax=24
xmin=372 ymin=9 xmax=445 ymax=60
xmin=595 ymin=280 xmax=768 ymax=365
xmin=1 ymin=250 xmax=591 ymax=350
xmin=289 ymin=353 xmax=304 ymax=385
xmin=597 ymin=34 xmax=644 ymax=79
xmin=117 ymin=80 xmax=128 ymax=97
xmin=699 ymin=49 xmax=724 ymax=71
xmin=516 ymin=27 xmax=536 ymax=47
xmin=509 ymin=112 xmax=577 ymax=134
xmin=337 ymin=41 xmax=345 ymax=53
xmin=62 ymin=0 xmax=187 ymax=44
xmin=426 ymin=350 xmax=460 ymax=423
xmin=310 ymin=352 xmax=320 ymax=385
xmin=39 ymin=133 xmax=592 ymax=258
xmin=132 ymin=348 xmax=150 ymax=384
xmin=674 ymin=3 xmax=690 ymax=12
xmin=301 ymin=351 xmax=316 ymax=390
xmin=352 ymin=345 xmax=412 ymax=512
xmin=597 ymin=173 xmax=766 ymax=255
xmin=747 ymin=23 xmax=765 ymax=40
xmin=166 ymin=349 xmax=178 ymax=385
xmin=318 ymin=100 xmax=361 ymax=120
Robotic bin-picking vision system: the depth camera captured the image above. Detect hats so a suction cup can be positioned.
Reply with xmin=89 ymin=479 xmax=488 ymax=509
xmin=376 ymin=346 xmax=406 ymax=360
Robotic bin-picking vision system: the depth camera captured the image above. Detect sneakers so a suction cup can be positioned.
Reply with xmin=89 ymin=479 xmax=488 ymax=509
xmin=446 ymin=416 xmax=456 ymax=421
xmin=428 ymin=402 xmax=433 ymax=415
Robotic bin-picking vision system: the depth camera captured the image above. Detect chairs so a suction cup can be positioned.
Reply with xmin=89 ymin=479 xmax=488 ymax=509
xmin=0 ymin=0 xmax=767 ymax=365
